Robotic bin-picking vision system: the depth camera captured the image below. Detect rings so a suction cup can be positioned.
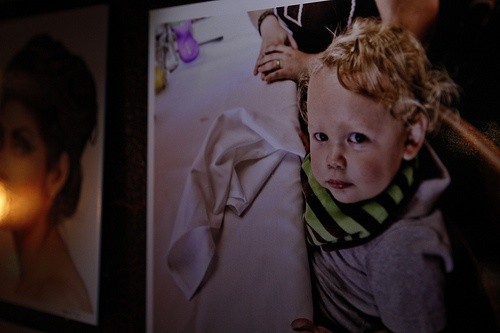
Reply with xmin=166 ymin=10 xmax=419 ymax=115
xmin=276 ymin=59 xmax=280 ymax=70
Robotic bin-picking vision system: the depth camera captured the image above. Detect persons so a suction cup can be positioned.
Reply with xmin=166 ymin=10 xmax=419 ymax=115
xmin=0 ymin=33 xmax=98 ymax=315
xmin=290 ymin=20 xmax=463 ymax=333
xmin=244 ymin=1 xmax=499 ymax=315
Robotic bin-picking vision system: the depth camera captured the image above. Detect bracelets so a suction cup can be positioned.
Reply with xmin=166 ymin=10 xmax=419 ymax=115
xmin=258 ymin=11 xmax=279 ymax=36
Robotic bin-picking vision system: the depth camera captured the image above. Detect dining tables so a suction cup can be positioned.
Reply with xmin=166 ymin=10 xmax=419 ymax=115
xmin=152 ymin=14 xmax=313 ymax=333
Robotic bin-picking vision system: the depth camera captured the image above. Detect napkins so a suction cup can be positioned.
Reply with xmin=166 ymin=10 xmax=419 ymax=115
xmin=165 ymin=107 xmax=306 ymax=301
